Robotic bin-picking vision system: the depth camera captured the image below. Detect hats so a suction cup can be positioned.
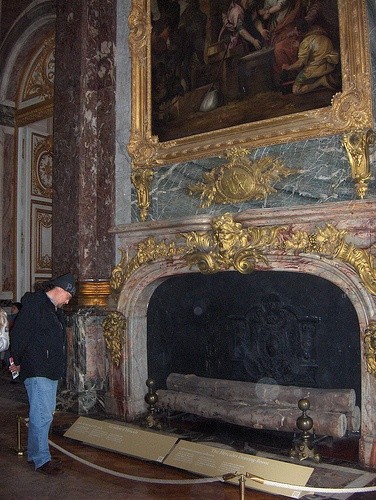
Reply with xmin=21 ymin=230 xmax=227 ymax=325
xmin=49 ymin=272 xmax=76 ymax=297
xmin=12 ymin=302 xmax=22 ymax=311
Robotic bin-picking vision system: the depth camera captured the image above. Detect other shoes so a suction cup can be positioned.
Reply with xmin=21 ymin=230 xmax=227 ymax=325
xmin=10 ymin=381 xmax=18 ymax=385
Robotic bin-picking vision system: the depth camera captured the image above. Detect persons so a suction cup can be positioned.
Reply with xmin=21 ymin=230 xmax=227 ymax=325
xmin=5 ymin=274 xmax=77 ymax=477
xmin=0 ymin=302 xmax=23 ymax=384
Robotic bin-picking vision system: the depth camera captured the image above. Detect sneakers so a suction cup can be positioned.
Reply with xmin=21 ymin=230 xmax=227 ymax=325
xmin=28 ymin=459 xmax=62 ymax=465
xmin=36 ymin=461 xmax=66 ymax=477
